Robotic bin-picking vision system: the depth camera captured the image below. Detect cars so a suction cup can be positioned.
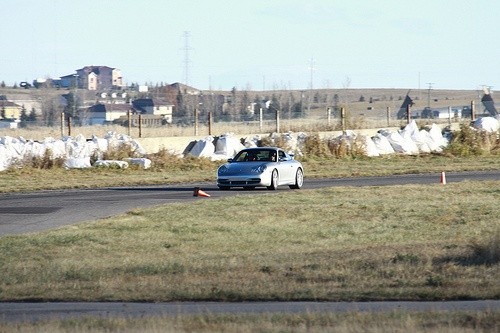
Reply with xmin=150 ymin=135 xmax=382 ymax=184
xmin=216 ymin=146 xmax=304 ymax=190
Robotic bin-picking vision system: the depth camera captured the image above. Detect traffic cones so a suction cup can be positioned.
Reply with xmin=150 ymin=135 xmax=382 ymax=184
xmin=192 ymin=186 xmax=211 ymax=198
xmin=440 ymin=171 xmax=447 ymax=185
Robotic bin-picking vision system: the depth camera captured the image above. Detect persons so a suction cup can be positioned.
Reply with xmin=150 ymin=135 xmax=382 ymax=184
xmin=267 ymin=150 xmax=277 ymax=161
xmin=248 ymin=150 xmax=257 ymax=159
